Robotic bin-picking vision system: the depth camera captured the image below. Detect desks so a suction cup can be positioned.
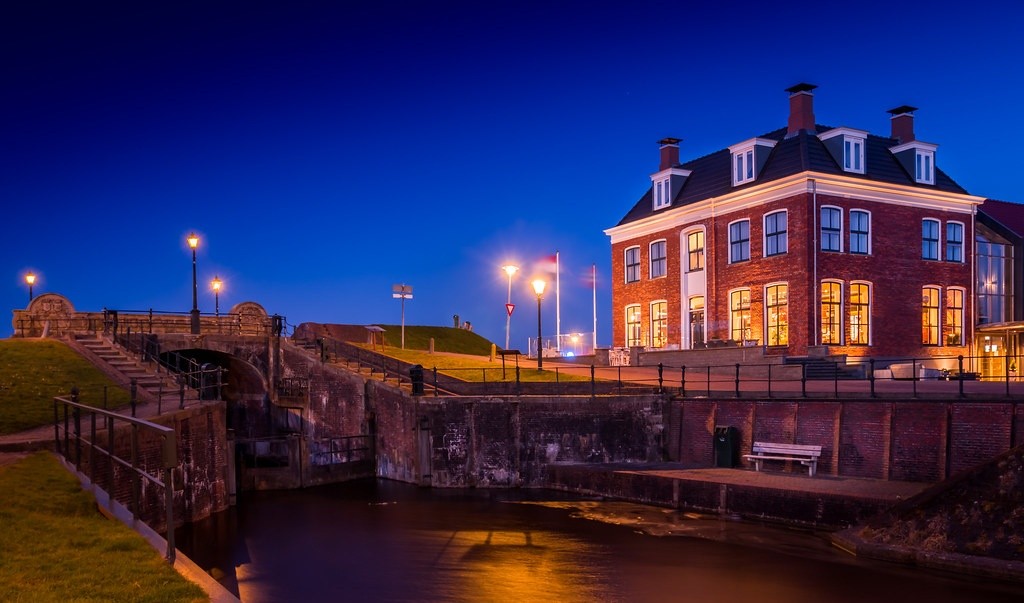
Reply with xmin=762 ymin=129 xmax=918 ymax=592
xmin=704 ymin=338 xmax=759 ymax=346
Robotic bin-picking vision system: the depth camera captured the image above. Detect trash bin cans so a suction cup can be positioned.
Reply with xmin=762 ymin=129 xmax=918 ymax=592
xmin=712 ymin=425 xmax=741 ymax=469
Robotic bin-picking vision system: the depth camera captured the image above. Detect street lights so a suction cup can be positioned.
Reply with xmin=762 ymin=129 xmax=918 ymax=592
xmin=187 ymin=232 xmax=205 ymax=334
xmin=25 ymin=270 xmax=39 ymax=302
xmin=498 ymin=258 xmax=520 ymax=349
xmin=209 ymin=277 xmax=222 ymax=319
xmin=529 ymin=272 xmax=552 ymax=370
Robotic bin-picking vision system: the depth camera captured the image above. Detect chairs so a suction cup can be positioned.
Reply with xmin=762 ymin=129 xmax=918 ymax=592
xmin=706 ymin=340 xmax=716 ymax=347
xmin=716 ymin=339 xmax=726 ymax=347
xmin=727 ymin=339 xmax=737 ymax=346
xmin=696 ymin=341 xmax=706 ymax=348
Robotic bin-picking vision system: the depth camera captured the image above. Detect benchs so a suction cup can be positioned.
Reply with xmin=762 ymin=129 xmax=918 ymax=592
xmin=742 ymin=442 xmax=822 ymax=477
